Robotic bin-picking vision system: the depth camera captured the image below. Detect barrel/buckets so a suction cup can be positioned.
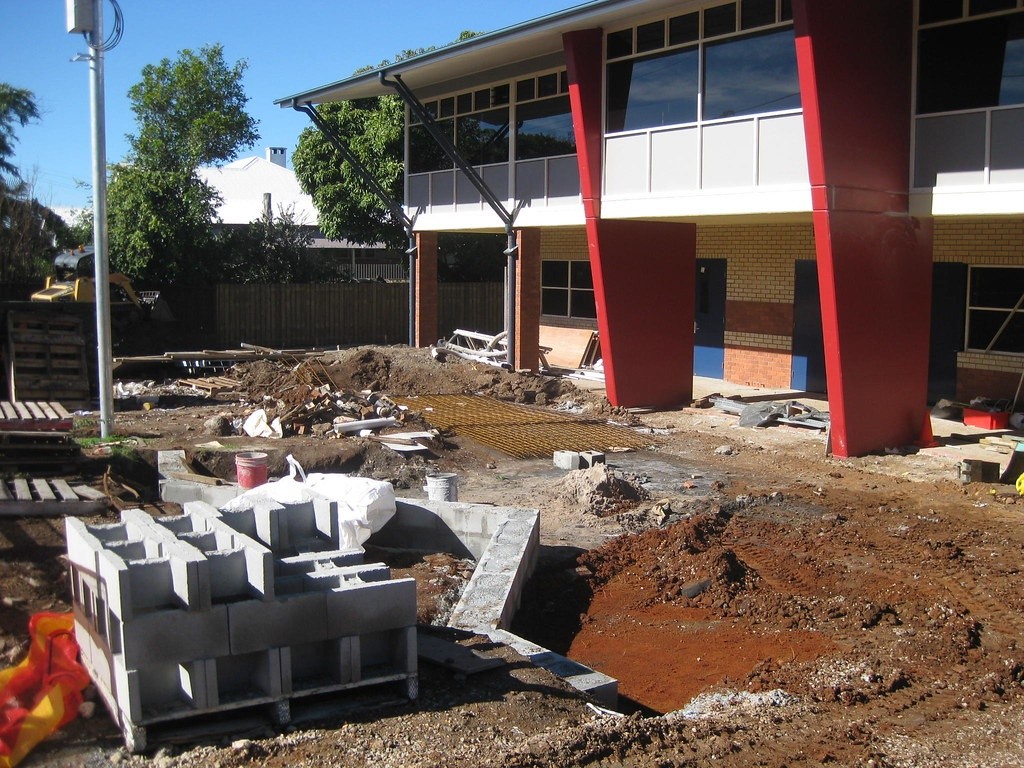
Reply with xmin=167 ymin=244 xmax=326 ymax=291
xmin=235 ymin=452 xmax=268 ymax=489
xmin=425 ymin=473 xmax=458 ymax=502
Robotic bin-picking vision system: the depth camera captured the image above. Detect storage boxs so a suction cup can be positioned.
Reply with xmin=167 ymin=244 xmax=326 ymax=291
xmin=552 ymin=449 xmax=579 ymax=469
xmin=579 ymin=450 xmax=605 ymax=468
xmin=963 ymin=407 xmax=1009 ymax=432
